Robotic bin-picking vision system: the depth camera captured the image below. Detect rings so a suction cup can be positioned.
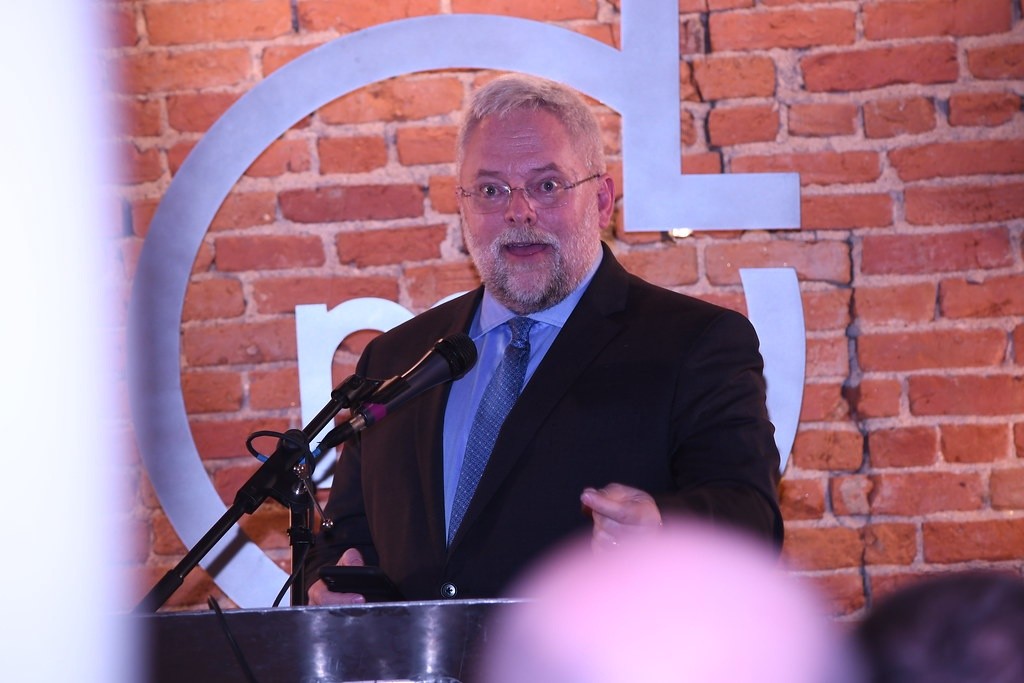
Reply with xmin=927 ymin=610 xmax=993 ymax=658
xmin=611 ymin=539 xmax=617 ymax=550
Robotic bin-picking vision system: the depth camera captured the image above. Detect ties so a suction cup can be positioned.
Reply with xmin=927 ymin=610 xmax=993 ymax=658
xmin=448 ymin=315 xmax=537 ymax=548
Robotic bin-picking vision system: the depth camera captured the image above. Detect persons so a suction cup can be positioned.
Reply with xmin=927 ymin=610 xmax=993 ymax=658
xmin=479 ymin=515 xmax=876 ymax=681
xmin=288 ymin=75 xmax=786 ymax=609
xmin=848 ymin=569 xmax=1023 ymax=683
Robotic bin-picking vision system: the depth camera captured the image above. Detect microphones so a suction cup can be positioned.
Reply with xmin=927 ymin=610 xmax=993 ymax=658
xmin=321 ymin=333 xmax=477 ymax=447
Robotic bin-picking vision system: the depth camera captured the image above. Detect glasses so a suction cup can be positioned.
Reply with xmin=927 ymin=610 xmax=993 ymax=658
xmin=456 ymin=171 xmax=610 ymax=217
